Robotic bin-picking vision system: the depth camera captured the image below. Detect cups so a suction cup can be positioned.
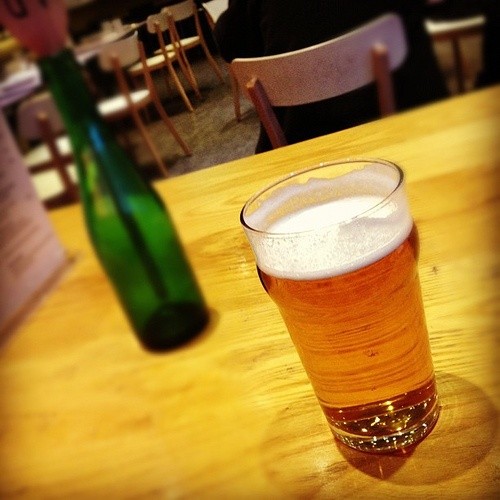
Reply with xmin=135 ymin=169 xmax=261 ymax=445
xmin=240 ymin=157 xmax=439 ymax=453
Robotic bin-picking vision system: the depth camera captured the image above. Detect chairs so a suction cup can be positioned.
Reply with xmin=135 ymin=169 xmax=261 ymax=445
xmin=232 ymin=15 xmax=409 ymax=151
xmin=425 ymin=15 xmax=488 ymax=97
xmin=15 ymin=0 xmax=230 ymax=211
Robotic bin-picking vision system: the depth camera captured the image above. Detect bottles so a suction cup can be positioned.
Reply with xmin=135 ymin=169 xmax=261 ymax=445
xmin=37 ymin=48 xmax=211 ymax=353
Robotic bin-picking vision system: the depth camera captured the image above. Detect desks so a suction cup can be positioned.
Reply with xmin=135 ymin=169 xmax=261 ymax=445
xmin=3 ymin=18 xmax=144 ymax=156
xmin=0 ymin=80 xmax=500 ymax=499
